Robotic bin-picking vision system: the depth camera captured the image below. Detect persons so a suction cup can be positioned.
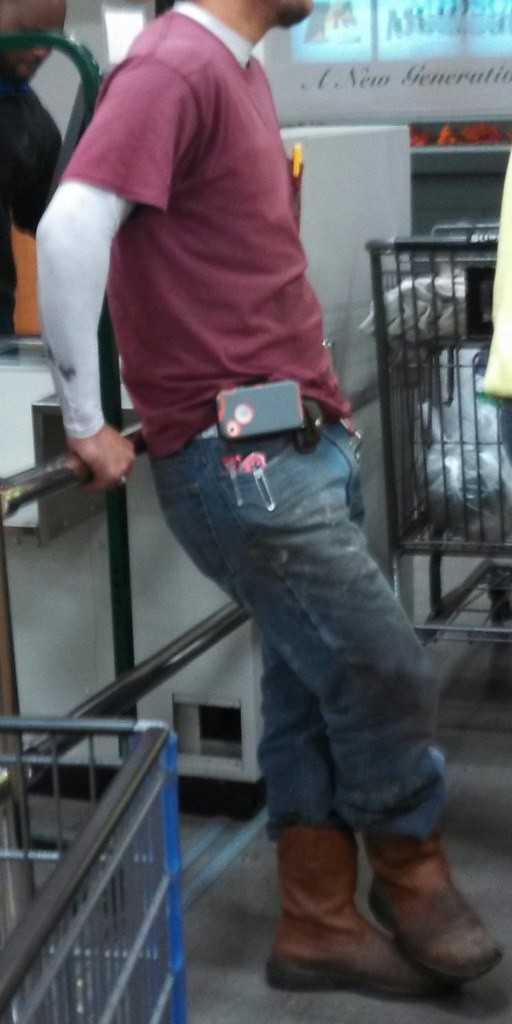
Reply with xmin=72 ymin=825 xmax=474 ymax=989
xmin=0 ymin=2 xmax=71 ymax=359
xmin=36 ymin=1 xmax=504 ymax=998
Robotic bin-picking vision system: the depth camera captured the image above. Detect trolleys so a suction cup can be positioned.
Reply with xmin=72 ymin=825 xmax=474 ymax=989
xmin=2 ymin=711 xmax=193 ymax=1024
xmin=366 ymin=219 xmax=512 ymax=638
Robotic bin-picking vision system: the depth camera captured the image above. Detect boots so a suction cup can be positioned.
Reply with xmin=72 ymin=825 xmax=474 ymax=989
xmin=263 ymin=825 xmax=457 ymax=997
xmin=362 ymin=822 xmax=502 ymax=978
